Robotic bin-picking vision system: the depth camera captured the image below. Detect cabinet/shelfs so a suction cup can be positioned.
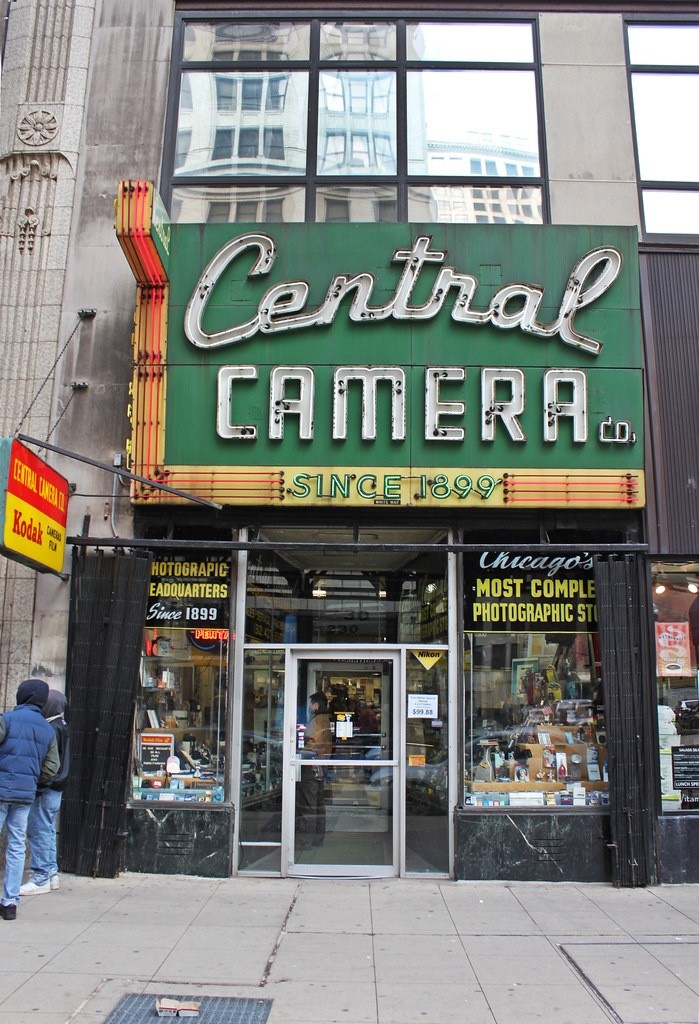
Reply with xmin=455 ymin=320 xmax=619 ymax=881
xmin=464 ymin=699 xmax=609 ymax=810
xmin=135 ymin=626 xmax=218 ymax=788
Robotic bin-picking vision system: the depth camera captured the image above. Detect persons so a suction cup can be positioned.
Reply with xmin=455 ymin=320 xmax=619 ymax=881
xmin=18 ymin=689 xmax=69 ymax=897
xmin=292 ymin=692 xmax=332 ymax=850
xmin=0 ymin=680 xmax=60 ymax=921
xmin=332 ymin=690 xmax=381 ymax=785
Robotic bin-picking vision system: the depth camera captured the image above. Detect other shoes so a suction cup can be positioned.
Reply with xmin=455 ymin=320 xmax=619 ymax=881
xmin=0 ymin=903 xmax=16 ymax=920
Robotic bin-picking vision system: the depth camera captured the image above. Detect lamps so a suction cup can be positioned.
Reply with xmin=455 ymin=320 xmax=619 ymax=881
xmin=687 ymin=581 xmax=699 ymax=593
xmin=655 ymin=582 xmax=666 ymax=594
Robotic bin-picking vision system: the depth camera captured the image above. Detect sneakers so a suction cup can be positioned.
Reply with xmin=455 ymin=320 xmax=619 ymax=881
xmin=19 ymin=879 xmax=51 ymax=895
xmin=50 ymin=875 xmax=59 ymax=889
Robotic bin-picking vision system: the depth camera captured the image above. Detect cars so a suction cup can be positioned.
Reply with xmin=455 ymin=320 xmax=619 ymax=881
xmin=552 ymin=698 xmax=594 ymax=727
xmin=664 ymin=686 xmax=699 ymax=736
xmin=171 ymin=728 xmax=325 ymax=814
xmin=368 ymin=727 xmax=587 ymax=818
